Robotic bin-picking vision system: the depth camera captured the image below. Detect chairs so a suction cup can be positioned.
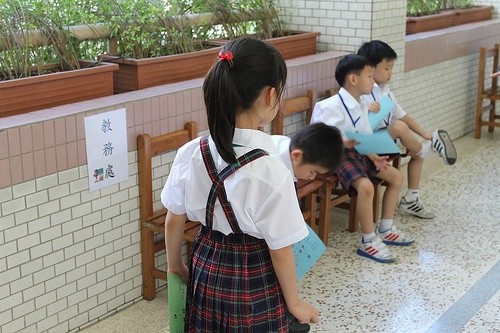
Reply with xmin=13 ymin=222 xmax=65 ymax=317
xmin=474 ymin=43 xmax=500 ymax=139
xmin=275 ymin=91 xmax=400 ymax=251
xmin=136 ymin=121 xmax=201 ymax=302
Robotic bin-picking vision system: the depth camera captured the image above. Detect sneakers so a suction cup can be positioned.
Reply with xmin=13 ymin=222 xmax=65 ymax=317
xmin=357 ymin=236 xmax=398 ymax=262
xmin=376 ymin=225 xmax=415 ymax=246
xmin=398 ymin=197 xmax=434 ymax=219
xmin=431 ymin=129 xmax=457 ymax=165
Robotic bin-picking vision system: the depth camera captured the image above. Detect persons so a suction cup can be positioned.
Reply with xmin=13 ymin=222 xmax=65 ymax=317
xmin=161 ymin=37 xmax=319 ymax=333
xmin=269 ymin=122 xmax=343 ymax=333
xmin=309 ymin=54 xmax=415 ymax=263
xmin=356 ymin=40 xmax=434 ymax=219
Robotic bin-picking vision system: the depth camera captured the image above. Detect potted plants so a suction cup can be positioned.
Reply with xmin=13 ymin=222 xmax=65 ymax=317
xmin=439 ymin=0 xmax=493 ymax=26
xmin=0 ymin=0 xmax=119 ymax=119
xmin=216 ymin=0 xmax=322 ymax=59
xmin=407 ymin=0 xmax=454 ymax=34
xmin=97 ymin=0 xmax=220 ymax=94
